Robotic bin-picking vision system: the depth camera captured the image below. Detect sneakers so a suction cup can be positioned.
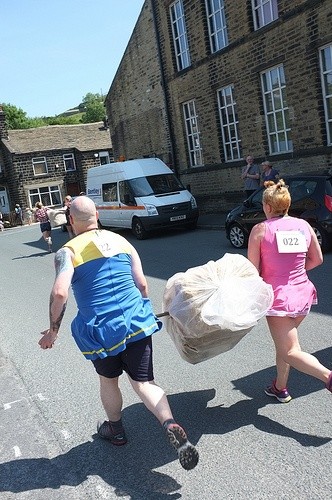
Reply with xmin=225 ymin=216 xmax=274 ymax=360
xmin=264 ymin=378 xmax=292 ymax=403
xmin=164 ymin=423 xmax=199 ymax=471
xmin=324 ymin=370 xmax=331 ymax=392
xmin=96 ymin=420 xmax=128 ymax=446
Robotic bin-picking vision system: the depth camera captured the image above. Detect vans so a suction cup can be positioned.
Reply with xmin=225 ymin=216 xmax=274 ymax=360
xmin=86 ymin=158 xmax=198 ymax=239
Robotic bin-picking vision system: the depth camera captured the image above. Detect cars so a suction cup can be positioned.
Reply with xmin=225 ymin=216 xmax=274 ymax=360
xmin=225 ymin=172 xmax=332 ymax=248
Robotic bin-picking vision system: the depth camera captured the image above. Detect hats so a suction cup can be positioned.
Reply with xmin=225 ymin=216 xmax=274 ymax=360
xmin=261 ymin=160 xmax=273 ymax=167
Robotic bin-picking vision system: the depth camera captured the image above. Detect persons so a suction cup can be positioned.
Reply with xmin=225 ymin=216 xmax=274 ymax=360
xmin=34 ymin=201 xmax=54 ymax=253
xmin=247 ymin=179 xmax=332 ymax=403
xmin=14 ymin=204 xmax=24 ymax=226
xmin=63 ymin=195 xmax=77 ymax=239
xmin=38 ymin=195 xmax=199 ymax=471
xmin=241 ymin=155 xmax=261 ymax=200
xmin=260 ymin=161 xmax=279 ymax=184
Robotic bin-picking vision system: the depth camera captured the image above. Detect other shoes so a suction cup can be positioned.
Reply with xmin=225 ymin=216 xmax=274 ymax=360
xmin=47 ymin=236 xmax=53 ymax=245
xmin=48 ymin=248 xmax=53 ymax=252
